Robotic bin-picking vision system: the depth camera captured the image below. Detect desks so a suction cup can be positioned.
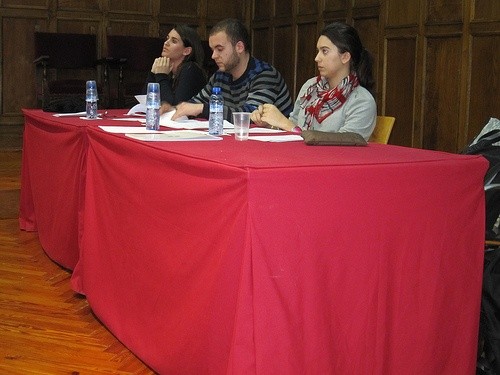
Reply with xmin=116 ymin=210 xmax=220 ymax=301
xmin=68 ymin=120 xmax=491 ymax=375
xmin=17 ymin=107 xmax=210 ymax=273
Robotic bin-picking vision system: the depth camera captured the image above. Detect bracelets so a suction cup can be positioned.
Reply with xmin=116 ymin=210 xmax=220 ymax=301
xmin=291 ymin=126 xmax=302 ymax=132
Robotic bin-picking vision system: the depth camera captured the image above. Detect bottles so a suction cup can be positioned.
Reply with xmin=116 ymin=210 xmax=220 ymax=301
xmin=87 ymin=82 xmax=97 ymax=118
xmin=209 ymin=87 xmax=224 ymax=136
xmin=146 ymin=83 xmax=160 ymax=130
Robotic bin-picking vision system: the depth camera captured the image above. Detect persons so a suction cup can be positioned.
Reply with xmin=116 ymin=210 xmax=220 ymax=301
xmin=142 ymin=26 xmax=207 ymax=105
xmin=159 ymin=18 xmax=294 ymax=125
xmin=250 ymin=22 xmax=377 ymax=142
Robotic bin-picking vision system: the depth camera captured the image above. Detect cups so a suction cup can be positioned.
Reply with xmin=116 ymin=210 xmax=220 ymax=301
xmin=146 ymin=83 xmax=161 ymax=108
xmin=232 ymin=112 xmax=253 ymax=140
xmin=86 ymin=81 xmax=99 ymax=100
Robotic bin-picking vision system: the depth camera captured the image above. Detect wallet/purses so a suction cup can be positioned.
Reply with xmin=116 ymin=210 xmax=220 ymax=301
xmin=300 ymin=130 xmax=368 ymax=146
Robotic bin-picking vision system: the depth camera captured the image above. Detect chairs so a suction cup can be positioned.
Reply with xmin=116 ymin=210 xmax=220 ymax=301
xmin=367 ymin=116 xmax=397 ymax=145
xmin=105 ymin=34 xmax=163 ymax=108
xmin=191 ymin=39 xmax=219 ymax=80
xmin=32 ymin=31 xmax=115 ymax=110
